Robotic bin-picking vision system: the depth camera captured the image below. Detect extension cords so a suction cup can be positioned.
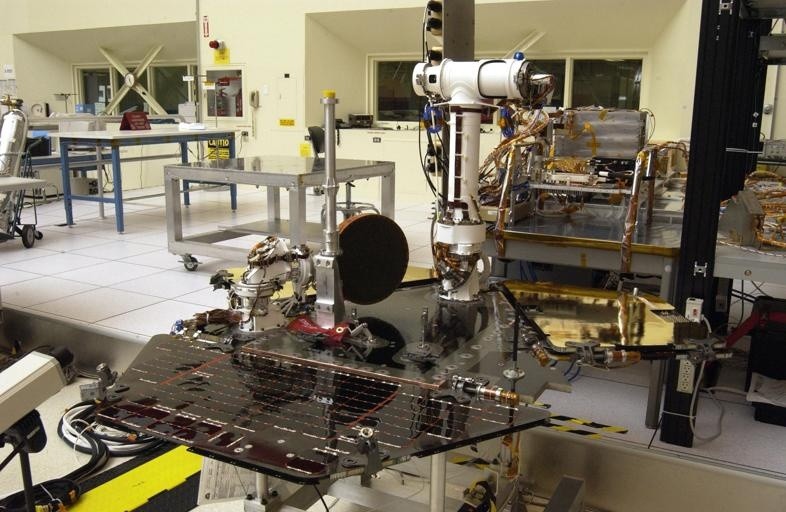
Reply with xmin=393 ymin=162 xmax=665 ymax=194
xmin=676 ymin=359 xmax=695 ymax=394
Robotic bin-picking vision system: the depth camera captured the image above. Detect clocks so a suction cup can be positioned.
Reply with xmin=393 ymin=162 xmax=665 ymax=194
xmin=124 ymin=72 xmax=136 ymax=86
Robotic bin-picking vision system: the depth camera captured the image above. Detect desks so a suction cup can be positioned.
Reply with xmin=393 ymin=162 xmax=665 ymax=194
xmin=46 ymin=129 xmax=241 ymax=233
xmin=475 ymin=198 xmax=683 ymax=430
xmin=164 ymin=154 xmax=395 ymax=275
